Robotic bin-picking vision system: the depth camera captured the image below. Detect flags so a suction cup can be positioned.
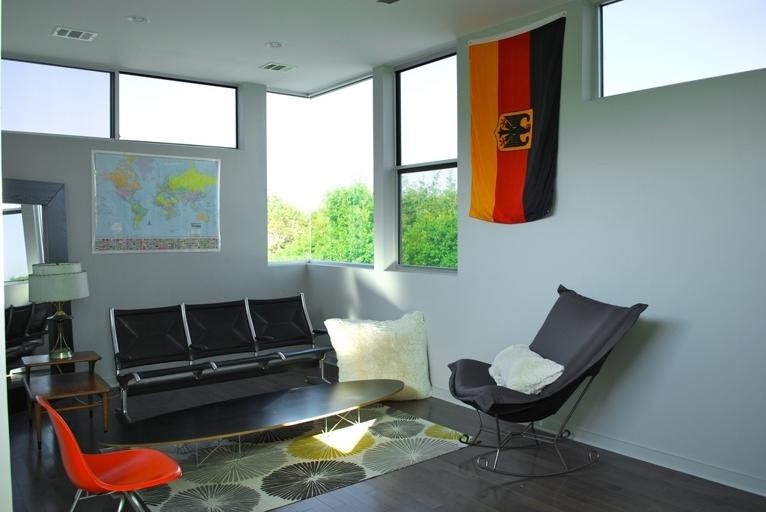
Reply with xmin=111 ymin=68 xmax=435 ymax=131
xmin=467 ymin=11 xmax=568 ymax=225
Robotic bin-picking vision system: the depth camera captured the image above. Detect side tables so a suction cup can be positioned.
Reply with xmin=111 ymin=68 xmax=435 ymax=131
xmin=22 ymin=350 xmax=112 ymax=450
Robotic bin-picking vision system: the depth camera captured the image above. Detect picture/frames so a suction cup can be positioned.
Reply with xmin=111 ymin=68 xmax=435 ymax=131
xmin=90 ymin=149 xmax=221 ymax=255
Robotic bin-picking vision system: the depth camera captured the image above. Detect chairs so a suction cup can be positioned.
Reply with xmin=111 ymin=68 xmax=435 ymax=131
xmin=109 ymin=293 xmax=335 ymax=424
xmin=4 ymin=301 xmax=51 ymax=375
xmin=35 ymin=394 xmax=183 ymax=512
xmin=447 ymin=285 xmax=649 ymax=478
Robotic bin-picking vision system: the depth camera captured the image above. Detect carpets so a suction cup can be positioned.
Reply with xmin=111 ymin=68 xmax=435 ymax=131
xmin=97 ymin=403 xmax=483 ymax=512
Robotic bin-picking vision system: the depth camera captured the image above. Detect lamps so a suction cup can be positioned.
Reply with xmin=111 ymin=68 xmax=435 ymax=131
xmin=28 ymin=263 xmax=90 ymax=359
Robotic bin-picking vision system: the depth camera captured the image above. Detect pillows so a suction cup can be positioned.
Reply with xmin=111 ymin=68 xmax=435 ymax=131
xmin=323 ymin=311 xmax=434 ymax=401
xmin=489 ymin=343 xmax=564 ymax=394
